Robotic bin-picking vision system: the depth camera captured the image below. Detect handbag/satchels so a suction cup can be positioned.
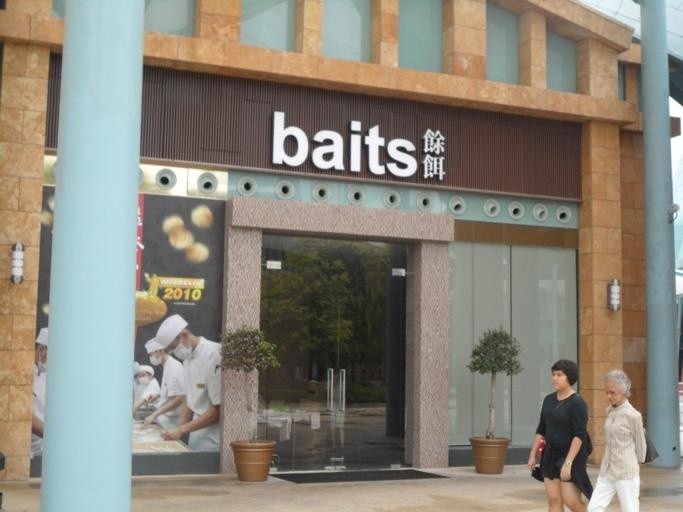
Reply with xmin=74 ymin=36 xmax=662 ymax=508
xmin=641 ymin=428 xmax=659 ymax=464
xmin=586 ymin=432 xmax=592 ymax=455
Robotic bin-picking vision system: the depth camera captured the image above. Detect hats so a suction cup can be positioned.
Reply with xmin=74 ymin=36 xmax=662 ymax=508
xmin=36 ymin=327 xmax=48 ymax=346
xmin=155 ymin=314 xmax=189 ymax=349
xmin=145 ymin=338 xmax=164 ymax=353
xmin=133 ymin=361 xmax=155 ymax=377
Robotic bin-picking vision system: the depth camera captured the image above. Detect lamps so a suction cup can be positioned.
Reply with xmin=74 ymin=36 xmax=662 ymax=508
xmin=606 ymin=277 xmax=622 ymax=311
xmin=9 ymin=244 xmax=28 ymax=283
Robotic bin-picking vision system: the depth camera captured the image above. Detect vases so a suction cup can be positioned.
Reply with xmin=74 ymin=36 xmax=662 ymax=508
xmin=359 ymin=437 xmax=400 ymax=470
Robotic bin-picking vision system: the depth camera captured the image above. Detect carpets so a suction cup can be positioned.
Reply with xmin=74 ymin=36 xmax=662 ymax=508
xmin=267 ymin=468 xmax=450 ymax=483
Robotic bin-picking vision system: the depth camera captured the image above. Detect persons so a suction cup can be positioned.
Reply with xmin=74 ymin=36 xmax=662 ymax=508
xmin=585 ymin=367 xmax=649 ymax=512
xmin=132 ymin=337 xmax=197 ymax=446
xmin=33 ymin=326 xmax=50 ymax=455
xmin=143 ymin=314 xmax=223 ymax=453
xmin=526 ymin=359 xmax=596 ymax=512
xmin=133 ymin=364 xmax=161 ymax=417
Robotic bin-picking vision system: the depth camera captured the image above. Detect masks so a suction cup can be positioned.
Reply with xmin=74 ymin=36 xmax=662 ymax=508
xmin=150 ymin=355 xmax=162 ymax=366
xmin=139 ymin=376 xmax=151 ymax=385
xmin=173 ymin=343 xmax=193 ymax=360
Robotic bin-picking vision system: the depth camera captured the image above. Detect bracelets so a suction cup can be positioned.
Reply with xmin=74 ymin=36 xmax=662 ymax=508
xmin=565 ymin=462 xmax=572 ymax=466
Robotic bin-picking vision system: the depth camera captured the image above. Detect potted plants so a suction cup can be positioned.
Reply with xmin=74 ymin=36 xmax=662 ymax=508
xmin=217 ymin=328 xmax=280 ymax=481
xmin=466 ymin=325 xmax=524 ymax=475
